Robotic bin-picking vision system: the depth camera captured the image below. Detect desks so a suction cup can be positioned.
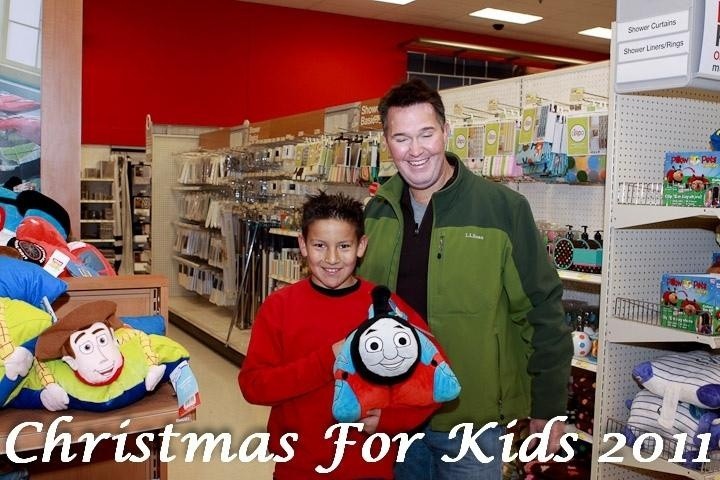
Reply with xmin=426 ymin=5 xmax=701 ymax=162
xmin=0 ymin=379 xmax=196 ymax=480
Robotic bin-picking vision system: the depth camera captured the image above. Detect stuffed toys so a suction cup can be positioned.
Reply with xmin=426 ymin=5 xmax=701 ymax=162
xmin=332 ymin=284 xmax=461 ymax=422
xmin=0 ymin=188 xmax=193 ymax=416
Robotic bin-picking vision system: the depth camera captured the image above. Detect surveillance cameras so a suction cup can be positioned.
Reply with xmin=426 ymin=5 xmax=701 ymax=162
xmin=493 ymin=24 xmax=504 ymax=31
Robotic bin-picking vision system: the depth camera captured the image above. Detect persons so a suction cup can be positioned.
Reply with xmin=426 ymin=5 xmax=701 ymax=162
xmin=236 ymin=190 xmax=450 ymax=480
xmin=353 ymin=79 xmax=571 ymax=479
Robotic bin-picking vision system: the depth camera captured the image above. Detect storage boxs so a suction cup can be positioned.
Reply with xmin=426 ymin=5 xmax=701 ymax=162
xmin=99 ymin=160 xmax=114 ymax=179
xmin=662 ymin=150 xmax=720 ymax=208
xmin=657 ymin=273 xmax=720 ymax=336
xmin=84 ymin=168 xmax=98 ymax=179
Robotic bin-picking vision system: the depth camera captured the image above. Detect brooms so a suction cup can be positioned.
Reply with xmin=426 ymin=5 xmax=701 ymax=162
xmin=223 ymin=217 xmax=282 ymax=347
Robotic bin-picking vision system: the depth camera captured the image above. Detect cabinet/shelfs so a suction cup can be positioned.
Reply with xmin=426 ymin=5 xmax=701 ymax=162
xmin=593 ymin=96 xmax=720 ymax=480
xmin=79 ymin=178 xmax=117 ymax=269
xmin=557 ymin=270 xmax=601 ymax=447
xmin=265 ymin=225 xmax=303 ymax=286
